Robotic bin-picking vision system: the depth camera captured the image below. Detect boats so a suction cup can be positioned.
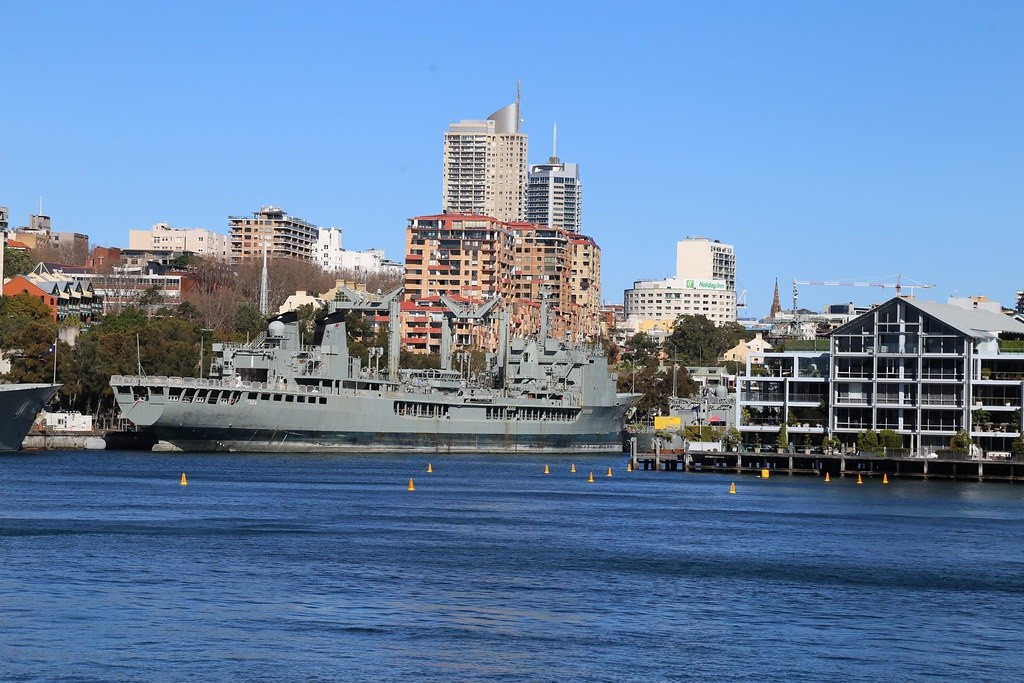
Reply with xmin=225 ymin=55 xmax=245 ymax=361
xmin=109 ymin=290 xmax=641 ymax=454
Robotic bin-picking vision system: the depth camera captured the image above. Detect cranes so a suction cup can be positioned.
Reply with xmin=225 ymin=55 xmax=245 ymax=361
xmin=794 ymin=271 xmax=935 ymax=297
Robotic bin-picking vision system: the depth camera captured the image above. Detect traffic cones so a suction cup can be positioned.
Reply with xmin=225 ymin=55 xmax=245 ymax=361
xmin=883 ymin=473 xmax=888 ymax=483
xmin=627 ymin=464 xmax=632 ymax=472
xmin=570 ymin=464 xmax=575 ymax=472
xmin=428 ymin=462 xmax=432 ymax=473
xmin=544 ymin=464 xmax=550 ymax=475
xmin=729 ymin=482 xmax=735 ymax=495
xmin=606 ymin=467 xmax=612 ymax=477
xmin=180 ymin=472 xmax=187 ymax=485
xmin=408 ymin=478 xmax=415 ymax=491
xmin=858 ymin=474 xmax=863 ymax=485
xmin=824 ymin=472 xmax=829 ymax=482
xmin=588 ymin=471 xmax=593 ymax=483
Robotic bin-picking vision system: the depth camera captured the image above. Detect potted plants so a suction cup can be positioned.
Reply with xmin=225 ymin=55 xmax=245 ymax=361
xmin=728 ymin=405 xmax=841 ymax=456
xmin=972 ymin=408 xmax=1022 ymax=433
xmin=974 ymin=395 xmax=984 ymax=405
xmin=1004 ymin=399 xmax=1012 ymax=407
xmin=981 ymin=367 xmax=991 ymax=380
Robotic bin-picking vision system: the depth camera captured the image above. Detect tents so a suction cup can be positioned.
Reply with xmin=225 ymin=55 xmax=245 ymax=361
xmin=705 ymin=415 xmax=725 ymax=426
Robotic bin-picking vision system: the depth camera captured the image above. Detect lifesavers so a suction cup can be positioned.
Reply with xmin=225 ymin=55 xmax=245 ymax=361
xmin=402 ymin=409 xmax=405 ymax=413
xmin=231 ymin=398 xmax=234 ymax=403
